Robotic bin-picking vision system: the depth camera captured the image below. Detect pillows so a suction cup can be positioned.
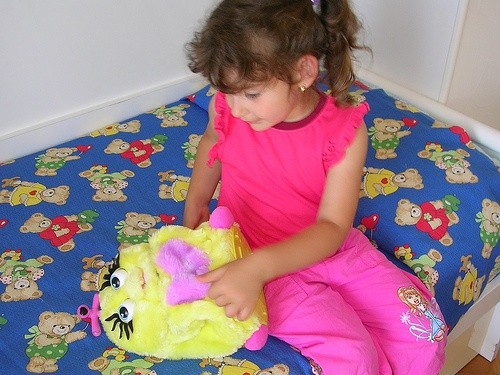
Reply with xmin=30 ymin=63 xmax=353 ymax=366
xmin=77 ymin=206 xmax=268 ymax=359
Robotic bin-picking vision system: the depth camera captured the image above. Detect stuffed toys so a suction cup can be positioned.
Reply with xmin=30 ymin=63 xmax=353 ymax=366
xmin=77 ymin=206 xmax=268 ymax=359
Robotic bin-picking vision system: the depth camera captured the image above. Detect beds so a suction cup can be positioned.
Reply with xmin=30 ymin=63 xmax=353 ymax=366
xmin=0 ymin=60 xmax=499 ymax=374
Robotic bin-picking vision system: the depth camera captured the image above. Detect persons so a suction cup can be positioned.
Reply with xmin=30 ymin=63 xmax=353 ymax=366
xmin=183 ymin=0 xmax=449 ymax=375
